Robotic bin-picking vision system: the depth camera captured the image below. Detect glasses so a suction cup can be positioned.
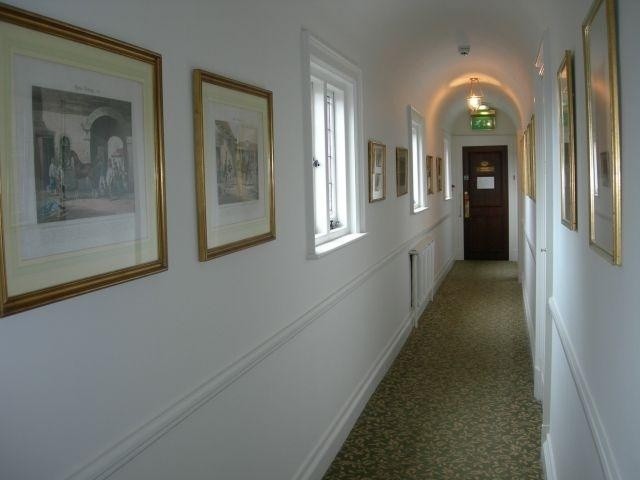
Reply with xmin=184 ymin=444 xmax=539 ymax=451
xmin=467 ymin=77 xmax=484 ymax=111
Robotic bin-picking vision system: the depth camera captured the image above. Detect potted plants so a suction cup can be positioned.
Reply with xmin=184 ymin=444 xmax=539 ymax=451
xmin=555 ymin=49 xmax=577 ymax=232
xmin=367 ymin=140 xmax=388 ymax=203
xmin=580 ymin=0 xmax=623 ymax=268
xmin=435 ymin=157 xmax=443 ymax=193
xmin=192 ymin=68 xmax=277 ymax=261
xmin=516 ymin=112 xmax=537 ymax=205
xmin=395 ymin=147 xmax=409 ymax=196
xmin=1 ymin=1 xmax=170 ymax=318
xmin=426 ymin=155 xmax=434 ymax=196
